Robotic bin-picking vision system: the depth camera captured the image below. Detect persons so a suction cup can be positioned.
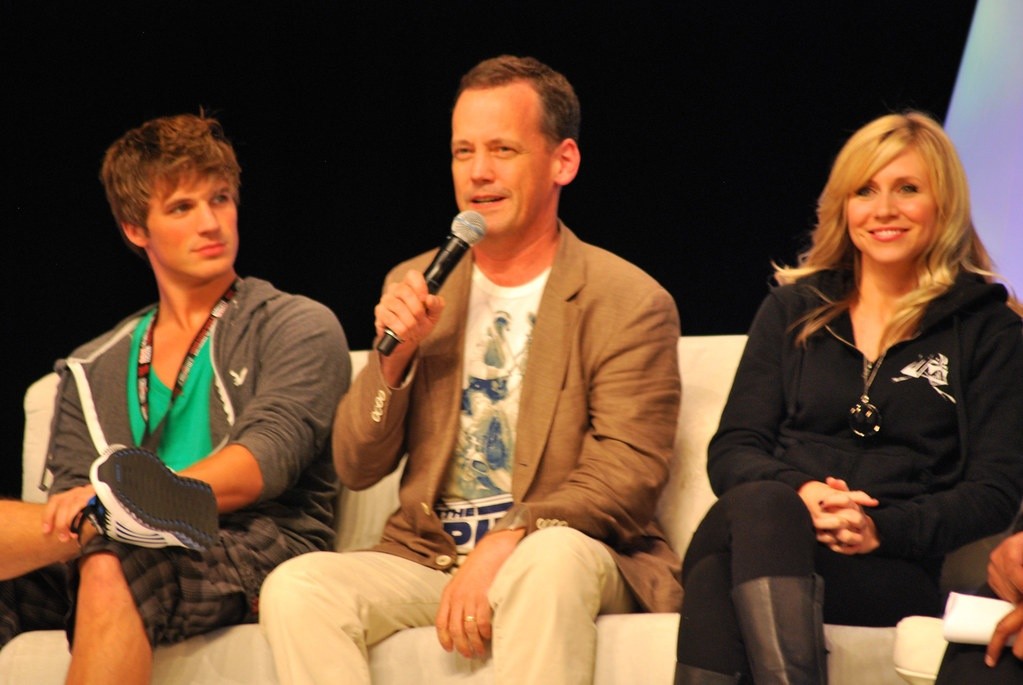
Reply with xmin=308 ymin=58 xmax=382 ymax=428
xmin=0 ymin=114 xmax=353 ymax=685
xmin=254 ymin=55 xmax=685 ymax=685
xmin=674 ymin=110 xmax=1022 ymax=685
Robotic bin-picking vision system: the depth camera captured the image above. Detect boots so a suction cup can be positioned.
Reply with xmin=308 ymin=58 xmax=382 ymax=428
xmin=731 ymin=573 xmax=828 ymax=684
xmin=674 ymin=661 xmax=737 ymax=685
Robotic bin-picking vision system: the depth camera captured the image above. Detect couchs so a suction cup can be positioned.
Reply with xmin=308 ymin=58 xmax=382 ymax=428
xmin=0 ymin=334 xmax=956 ymax=685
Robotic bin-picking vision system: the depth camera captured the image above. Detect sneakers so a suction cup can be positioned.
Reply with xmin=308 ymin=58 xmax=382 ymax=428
xmin=70 ymin=444 xmax=219 ymax=553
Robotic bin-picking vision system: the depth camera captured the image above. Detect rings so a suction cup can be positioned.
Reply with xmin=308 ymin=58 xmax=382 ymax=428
xmin=463 ymin=616 xmax=476 ymax=622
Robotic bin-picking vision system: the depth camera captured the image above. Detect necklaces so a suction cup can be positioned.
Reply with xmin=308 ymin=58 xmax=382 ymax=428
xmin=849 ymin=356 xmax=886 ymax=438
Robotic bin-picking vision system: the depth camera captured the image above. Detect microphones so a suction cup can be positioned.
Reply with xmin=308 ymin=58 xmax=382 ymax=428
xmin=376 ymin=211 xmax=488 ymax=357
